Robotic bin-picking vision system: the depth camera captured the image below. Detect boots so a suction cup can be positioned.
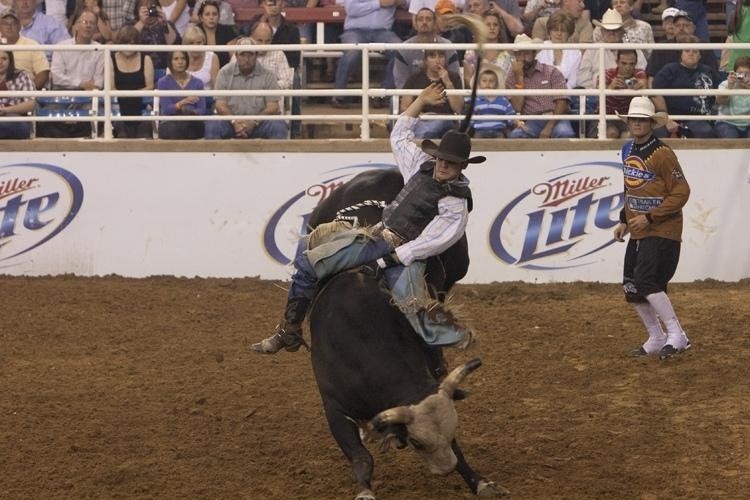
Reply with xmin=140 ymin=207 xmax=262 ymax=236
xmin=433 ymin=363 xmax=467 ymax=401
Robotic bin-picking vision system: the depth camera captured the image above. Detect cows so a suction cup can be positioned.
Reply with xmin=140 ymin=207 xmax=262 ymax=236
xmin=306 ymin=14 xmax=510 ymax=500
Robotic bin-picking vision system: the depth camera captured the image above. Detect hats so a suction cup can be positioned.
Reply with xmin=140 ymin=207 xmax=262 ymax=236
xmin=434 ymin=0 xmax=456 ymax=13
xmin=236 ymin=38 xmax=256 ymax=56
xmin=507 ymin=34 xmax=544 ymax=56
xmin=469 ymin=63 xmax=507 ymax=90
xmin=421 ymin=130 xmax=487 ymax=164
xmin=615 ymin=98 xmax=669 ymax=128
xmin=661 ymin=7 xmax=692 ymax=23
xmin=2 ymin=8 xmax=19 ymax=19
xmin=591 ymin=8 xmax=635 ymax=30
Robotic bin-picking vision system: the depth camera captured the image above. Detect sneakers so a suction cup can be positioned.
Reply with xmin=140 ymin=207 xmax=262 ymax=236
xmin=661 ymin=329 xmax=691 ymax=357
xmin=637 ymin=333 xmax=667 ymax=357
xmin=252 ymin=296 xmax=309 ymax=354
xmin=332 ymin=97 xmax=348 ymax=108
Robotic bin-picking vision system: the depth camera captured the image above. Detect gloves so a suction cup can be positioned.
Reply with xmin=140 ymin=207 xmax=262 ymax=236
xmin=361 ymin=254 xmax=398 ymax=274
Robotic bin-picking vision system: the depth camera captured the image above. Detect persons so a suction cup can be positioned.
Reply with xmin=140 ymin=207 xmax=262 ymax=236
xmin=249 ymin=83 xmax=487 ymax=402
xmin=0 ymin=0 xmax=750 ymax=139
xmin=612 ymin=95 xmax=695 ymax=362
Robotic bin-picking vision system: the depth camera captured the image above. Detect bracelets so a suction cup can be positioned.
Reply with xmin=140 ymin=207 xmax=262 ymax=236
xmin=645 ymin=212 xmax=654 ymax=226
xmin=375 ymin=254 xmax=399 ymax=269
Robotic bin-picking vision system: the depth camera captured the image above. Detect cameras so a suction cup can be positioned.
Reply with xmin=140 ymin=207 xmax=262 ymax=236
xmin=148 ymin=7 xmax=157 ymax=17
xmin=624 ymin=79 xmax=635 ymax=88
xmin=734 ymin=73 xmax=746 ymax=82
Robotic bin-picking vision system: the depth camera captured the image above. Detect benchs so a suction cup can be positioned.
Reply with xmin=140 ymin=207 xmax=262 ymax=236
xmin=226 ymin=2 xmax=748 ymax=138
